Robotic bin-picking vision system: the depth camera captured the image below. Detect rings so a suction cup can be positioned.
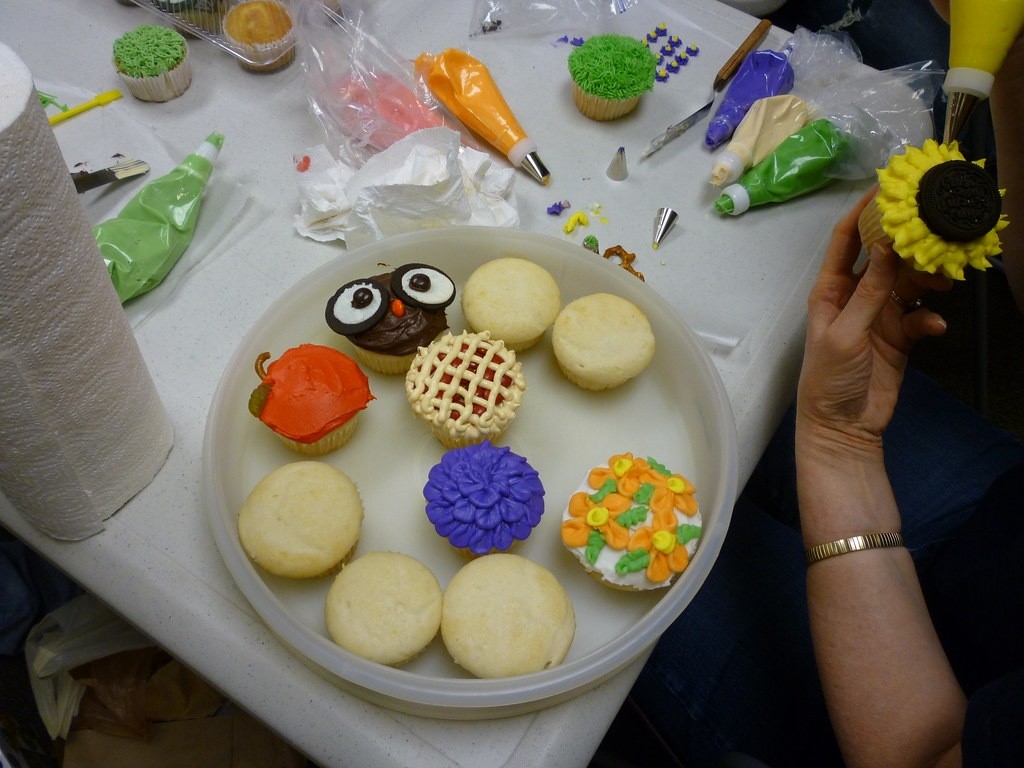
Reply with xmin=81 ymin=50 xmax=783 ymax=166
xmin=890 ymin=289 xmax=921 ymax=313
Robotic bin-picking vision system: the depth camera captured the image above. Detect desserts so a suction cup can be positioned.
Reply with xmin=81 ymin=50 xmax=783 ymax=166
xmin=111 ymin=2 xmax=1011 ymax=680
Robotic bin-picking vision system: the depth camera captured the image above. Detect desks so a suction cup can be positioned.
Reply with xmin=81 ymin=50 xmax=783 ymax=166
xmin=5 ymin=3 xmax=932 ymax=768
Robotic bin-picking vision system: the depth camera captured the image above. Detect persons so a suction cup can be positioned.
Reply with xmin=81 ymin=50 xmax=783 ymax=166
xmin=579 ymin=0 xmax=1024 ymax=767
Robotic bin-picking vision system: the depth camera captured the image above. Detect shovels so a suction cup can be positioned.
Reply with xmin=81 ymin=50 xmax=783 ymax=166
xmin=640 ymin=20 xmax=772 ymax=165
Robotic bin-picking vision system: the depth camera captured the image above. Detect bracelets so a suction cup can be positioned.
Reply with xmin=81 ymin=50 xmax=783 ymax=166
xmin=804 ymin=529 xmax=904 ymax=568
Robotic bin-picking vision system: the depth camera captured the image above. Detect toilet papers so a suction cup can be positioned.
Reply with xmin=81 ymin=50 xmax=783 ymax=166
xmin=0 ymin=42 xmax=179 ymax=547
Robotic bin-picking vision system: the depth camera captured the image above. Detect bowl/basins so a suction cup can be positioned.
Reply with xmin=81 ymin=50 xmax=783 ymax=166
xmin=200 ymin=224 xmax=739 ymax=722
